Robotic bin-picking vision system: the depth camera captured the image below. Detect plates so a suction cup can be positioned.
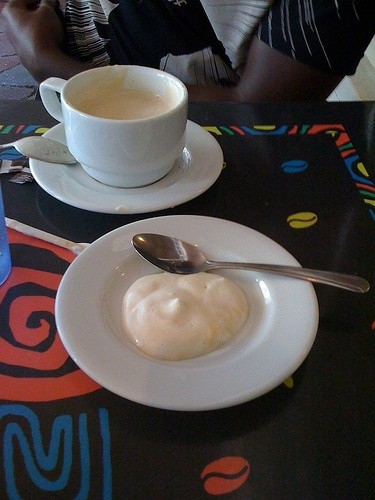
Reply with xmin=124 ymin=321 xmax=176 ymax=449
xmin=54 ymin=216 xmax=318 ymax=411
xmin=29 ymin=118 xmax=223 ymax=214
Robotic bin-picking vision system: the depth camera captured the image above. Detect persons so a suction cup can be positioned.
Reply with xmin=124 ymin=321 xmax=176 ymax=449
xmin=1 ymin=0 xmax=375 ymax=105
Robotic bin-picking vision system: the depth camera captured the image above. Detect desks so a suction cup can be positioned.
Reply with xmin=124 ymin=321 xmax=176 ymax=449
xmin=0 ymin=99 xmax=375 ymax=500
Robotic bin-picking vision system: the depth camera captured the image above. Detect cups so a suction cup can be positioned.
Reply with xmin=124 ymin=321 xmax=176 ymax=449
xmin=0 ymin=185 xmax=11 ymax=287
xmin=39 ymin=66 xmax=188 ymax=187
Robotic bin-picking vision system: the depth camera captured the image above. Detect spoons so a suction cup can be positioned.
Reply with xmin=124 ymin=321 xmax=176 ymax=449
xmin=0 ymin=136 xmax=77 ymax=165
xmin=131 ymin=233 xmax=369 ymax=293
